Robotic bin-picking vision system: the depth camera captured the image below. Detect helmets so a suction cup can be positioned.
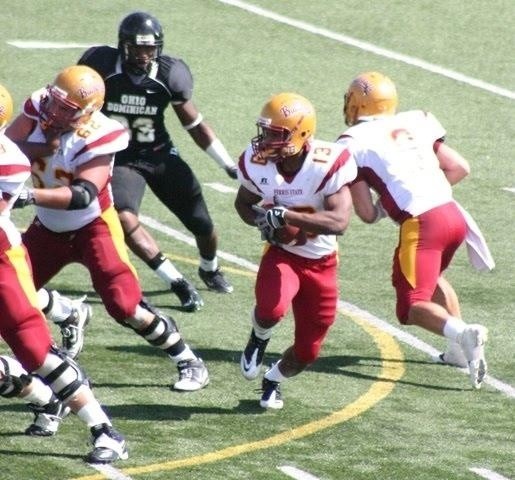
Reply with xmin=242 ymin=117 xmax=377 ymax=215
xmin=344 ymin=72 xmax=398 ymax=124
xmin=118 ymin=13 xmax=163 ymax=62
xmin=252 ymin=93 xmax=316 ymax=159
xmin=40 ymin=65 xmax=105 ymax=130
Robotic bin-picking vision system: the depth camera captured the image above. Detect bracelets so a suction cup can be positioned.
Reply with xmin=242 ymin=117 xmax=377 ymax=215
xmin=205 ymin=139 xmax=236 ymax=171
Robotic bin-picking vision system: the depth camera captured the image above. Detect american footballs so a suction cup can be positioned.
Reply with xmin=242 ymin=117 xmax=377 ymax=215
xmin=261 ymin=203 xmax=306 ymax=246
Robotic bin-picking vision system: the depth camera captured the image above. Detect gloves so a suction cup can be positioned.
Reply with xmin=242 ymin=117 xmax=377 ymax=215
xmin=251 ymin=205 xmax=288 ymax=238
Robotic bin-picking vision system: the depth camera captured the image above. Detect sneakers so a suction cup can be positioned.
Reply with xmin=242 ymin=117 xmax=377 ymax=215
xmin=174 ymin=357 xmax=209 ymax=390
xmin=240 ymin=327 xmax=283 ymax=409
xmin=25 ymin=400 xmax=71 ymax=435
xmin=432 ymin=324 xmax=487 ymax=389
xmin=55 ymin=301 xmax=92 ymax=360
xmin=172 ymin=267 xmax=233 ymax=311
xmin=88 ymin=423 xmax=128 ymax=462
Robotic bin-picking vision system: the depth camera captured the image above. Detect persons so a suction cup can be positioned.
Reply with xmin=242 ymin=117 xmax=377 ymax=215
xmin=77 ymin=12 xmax=238 ymax=312
xmin=0 ymin=84 xmax=92 ymax=359
xmin=5 ymin=65 xmax=209 ymax=391
xmin=334 ymin=73 xmax=487 ymax=391
xmin=235 ymin=92 xmax=359 ymax=410
xmin=0 ymin=131 xmax=128 ymax=461
